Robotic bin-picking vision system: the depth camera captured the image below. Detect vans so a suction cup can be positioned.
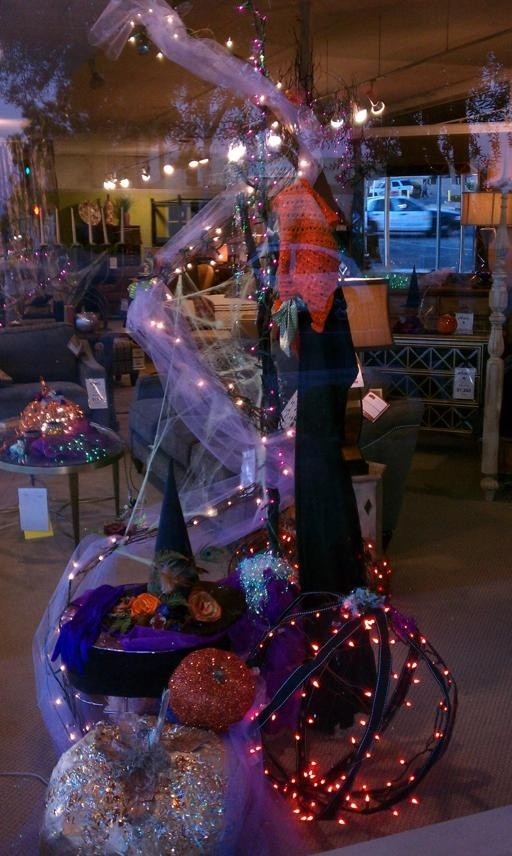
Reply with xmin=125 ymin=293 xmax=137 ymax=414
xmin=369 ymin=180 xmax=413 ymax=194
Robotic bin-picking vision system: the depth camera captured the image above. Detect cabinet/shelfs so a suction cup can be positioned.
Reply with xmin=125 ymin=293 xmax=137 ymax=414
xmin=72 ymin=226 xmax=142 ymax=319
xmin=357 ymin=335 xmax=489 ymax=450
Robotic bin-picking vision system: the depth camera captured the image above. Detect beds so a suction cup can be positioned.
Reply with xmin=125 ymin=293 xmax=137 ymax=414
xmin=163 ymin=293 xmax=259 ymax=343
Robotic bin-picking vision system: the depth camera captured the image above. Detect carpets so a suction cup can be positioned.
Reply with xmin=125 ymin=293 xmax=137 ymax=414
xmin=0 ymin=321 xmax=512 ymax=856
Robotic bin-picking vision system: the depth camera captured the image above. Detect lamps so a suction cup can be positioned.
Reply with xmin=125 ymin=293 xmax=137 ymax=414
xmin=187 ymin=147 xmax=198 ymax=167
xmin=139 ymin=40 xmax=152 ymax=54
xmin=129 ymin=32 xmax=143 ymax=42
xmin=331 ymin=115 xmax=346 ymax=128
xmin=141 ymin=164 xmax=151 ymax=181
xmin=227 ymin=35 xmax=231 ymax=48
xmin=479 ymin=134 xmax=512 ymax=502
xmin=172 ymin=0 xmax=178 ymax=10
xmin=353 ymin=101 xmax=366 ymax=122
xmin=104 ymin=173 xmax=117 ymax=190
xmin=86 ymin=56 xmax=105 ymax=90
xmin=120 ymin=175 xmax=129 ymax=187
xmin=337 ymin=277 xmax=394 ymax=475
xmin=365 ymin=91 xmax=385 ymax=115
xmin=460 ymin=192 xmax=512 ymax=290
xmin=198 ymin=143 xmax=211 ymax=164
xmin=163 ymin=155 xmax=175 ymax=175
xmin=216 ymin=244 xmax=228 ymax=264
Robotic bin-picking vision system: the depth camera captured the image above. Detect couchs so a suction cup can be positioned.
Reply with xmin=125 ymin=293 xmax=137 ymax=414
xmin=113 ymin=338 xmax=141 ymax=386
xmin=359 ymin=400 xmax=425 ymax=554
xmin=0 ymin=322 xmax=120 ymax=433
xmin=129 ymin=340 xmax=299 ymax=497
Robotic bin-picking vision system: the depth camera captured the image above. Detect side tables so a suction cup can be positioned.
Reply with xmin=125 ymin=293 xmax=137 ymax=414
xmin=351 ymin=461 xmax=386 ymax=561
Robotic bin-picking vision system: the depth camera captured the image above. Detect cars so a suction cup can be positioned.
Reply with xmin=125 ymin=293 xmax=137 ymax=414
xmin=366 ymin=197 xmax=461 ymax=233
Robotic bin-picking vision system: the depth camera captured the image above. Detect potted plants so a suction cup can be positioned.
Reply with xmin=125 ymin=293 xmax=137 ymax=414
xmin=111 ymin=193 xmax=134 ymax=225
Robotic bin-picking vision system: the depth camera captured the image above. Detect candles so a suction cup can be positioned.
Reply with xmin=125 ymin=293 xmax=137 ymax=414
xmin=39 ymin=207 xmax=44 ymax=243
xmin=55 ymin=207 xmax=61 ymax=242
xmin=101 ymin=207 xmax=108 ymax=244
xmin=186 ymin=206 xmax=192 ymax=223
xmin=121 ymin=206 xmax=124 ymax=242
xmin=70 ymin=207 xmax=77 ymax=244
xmin=87 ymin=206 xmax=93 ymax=244
